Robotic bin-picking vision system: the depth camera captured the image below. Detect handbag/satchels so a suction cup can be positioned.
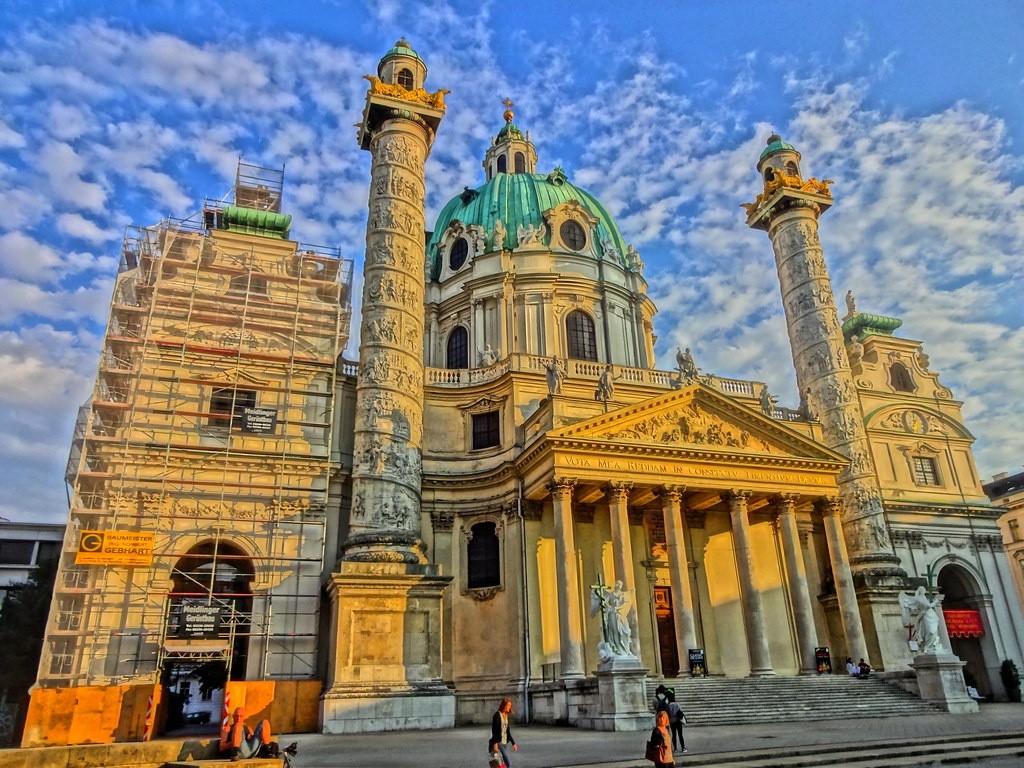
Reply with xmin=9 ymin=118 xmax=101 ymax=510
xmin=645 ymin=740 xmax=665 ymax=763
xmin=677 ymin=709 xmax=688 ymax=726
xmin=488 ymin=750 xmax=508 ymax=768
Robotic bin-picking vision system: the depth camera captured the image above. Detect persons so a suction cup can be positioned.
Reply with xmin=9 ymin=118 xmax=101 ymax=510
xmin=899 ymin=586 xmax=952 ymax=654
xmin=477 ymin=345 xmax=499 ymax=367
xmin=626 ymin=244 xmax=643 ymax=273
xmin=220 ymin=708 xmax=277 ymax=761
xmin=598 ymin=365 xmax=622 ymax=400
xmin=488 ymin=698 xmax=519 ymax=768
xmin=846 ymin=657 xmax=860 ymax=676
xmin=676 ymin=347 xmax=699 ymax=380
xmin=522 ymin=222 xmax=544 ymax=245
xmin=759 ymin=385 xmax=774 ymax=416
xmin=846 ymin=290 xmax=855 ymax=313
xmin=492 ymin=218 xmax=506 ymax=246
xmin=667 ymin=692 xmax=688 ymax=753
xmin=477 ymin=224 xmax=486 ymax=247
xmin=653 ymin=685 xmax=671 ymax=738
xmin=540 ymin=356 xmax=567 ymax=395
xmin=651 ymin=711 xmax=677 ymax=768
xmin=591 ymin=580 xmax=637 ymax=660
xmin=858 ymin=658 xmax=870 ymax=680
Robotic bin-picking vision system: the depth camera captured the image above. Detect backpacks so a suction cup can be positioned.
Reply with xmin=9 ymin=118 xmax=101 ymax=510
xmin=655 ymin=695 xmax=671 ymax=719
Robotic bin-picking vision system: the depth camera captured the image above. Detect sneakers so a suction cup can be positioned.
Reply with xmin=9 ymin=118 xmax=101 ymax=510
xmin=230 ymin=748 xmax=242 ymax=761
xmin=673 ymin=749 xmax=678 ymax=752
xmin=261 ymin=745 xmax=272 ymax=759
xmin=682 ymin=748 xmax=688 ymax=753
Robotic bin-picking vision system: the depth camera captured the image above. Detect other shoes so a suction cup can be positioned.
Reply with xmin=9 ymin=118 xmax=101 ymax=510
xmin=858 ymin=675 xmax=869 ymax=680
